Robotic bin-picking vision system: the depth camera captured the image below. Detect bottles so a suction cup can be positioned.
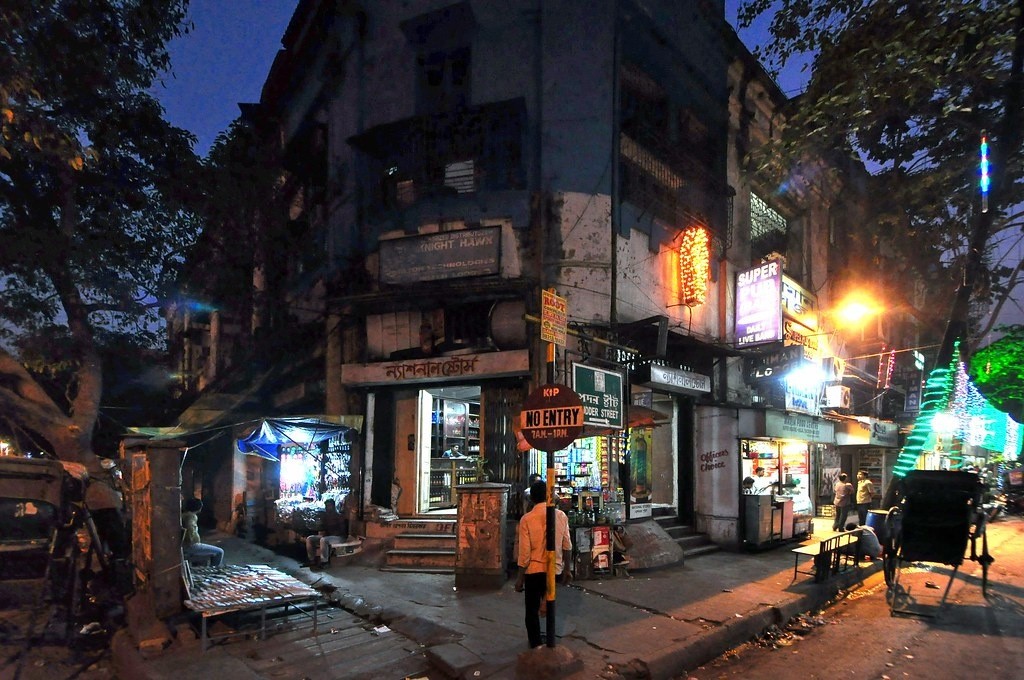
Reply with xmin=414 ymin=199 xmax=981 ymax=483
xmin=468 ymin=417 xmax=480 ymax=451
xmin=429 ymin=471 xmax=476 ymax=503
xmin=635 ymin=426 xmax=647 ymax=479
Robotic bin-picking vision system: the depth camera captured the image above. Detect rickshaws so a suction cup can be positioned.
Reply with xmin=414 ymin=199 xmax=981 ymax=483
xmin=882 ymin=471 xmax=995 ymax=599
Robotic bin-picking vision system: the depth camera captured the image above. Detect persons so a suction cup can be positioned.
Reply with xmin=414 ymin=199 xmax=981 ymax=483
xmin=754 ymin=467 xmax=764 ymax=488
xmin=523 ymin=473 xmax=542 ymax=514
xmin=181 ymin=498 xmax=224 ymax=566
xmin=856 ymin=470 xmax=876 ymax=526
xmin=442 ymin=443 xmax=463 ymax=458
xmin=306 ymin=499 xmax=348 ymax=569
xmin=846 ymin=523 xmax=880 ymax=561
xmin=742 ymin=477 xmax=754 ymax=495
xmin=515 ymin=480 xmax=572 ymax=649
xmin=833 ymin=472 xmax=855 ymax=532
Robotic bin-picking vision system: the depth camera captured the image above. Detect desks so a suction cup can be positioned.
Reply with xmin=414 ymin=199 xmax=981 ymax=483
xmin=184 ymin=564 xmax=321 ymax=652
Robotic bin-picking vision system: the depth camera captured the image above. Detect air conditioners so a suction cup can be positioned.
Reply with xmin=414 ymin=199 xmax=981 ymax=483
xmin=823 ymin=357 xmax=842 ymax=381
xmin=826 ymin=385 xmax=850 ymax=409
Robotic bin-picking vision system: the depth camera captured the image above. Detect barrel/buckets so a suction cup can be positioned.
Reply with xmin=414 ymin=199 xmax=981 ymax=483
xmin=865 ymin=510 xmax=888 ymax=545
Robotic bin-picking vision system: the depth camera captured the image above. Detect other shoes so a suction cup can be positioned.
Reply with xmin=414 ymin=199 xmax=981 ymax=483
xmin=839 ymin=530 xmax=846 ymax=532
xmin=538 ymin=601 xmax=547 ymax=616
xmin=535 ymin=645 xmax=543 ymax=650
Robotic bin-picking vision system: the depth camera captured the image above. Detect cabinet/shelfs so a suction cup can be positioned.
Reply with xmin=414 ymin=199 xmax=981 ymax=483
xmin=431 ymin=400 xmax=479 ymax=458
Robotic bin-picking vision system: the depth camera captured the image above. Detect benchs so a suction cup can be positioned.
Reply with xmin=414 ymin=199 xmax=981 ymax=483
xmin=791 ymin=529 xmax=862 ymax=582
